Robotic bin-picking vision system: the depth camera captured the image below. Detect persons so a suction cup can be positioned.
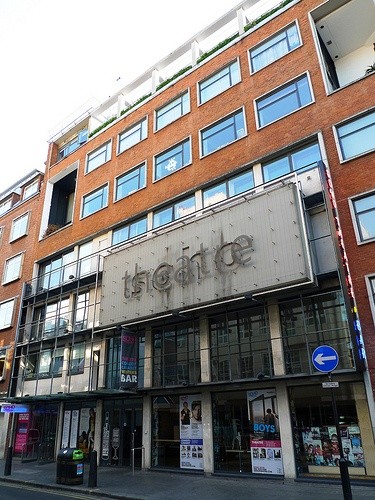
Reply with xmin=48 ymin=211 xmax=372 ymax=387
xmin=84 ymin=408 xmax=96 ymax=454
xmin=78 ymin=430 xmax=87 ymax=452
xmin=180 ymin=400 xmax=191 ymax=425
xmin=252 ymin=447 xmax=282 ymax=461
xmin=304 ymin=427 xmax=364 ymax=468
xmin=191 ymin=400 xmax=202 ymax=423
xmin=180 ymin=445 xmax=203 ymax=458
xmin=262 ymin=408 xmax=276 ymax=440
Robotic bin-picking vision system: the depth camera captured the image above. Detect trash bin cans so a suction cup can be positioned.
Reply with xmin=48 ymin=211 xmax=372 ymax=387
xmin=56 ymin=447 xmax=85 ymax=485
